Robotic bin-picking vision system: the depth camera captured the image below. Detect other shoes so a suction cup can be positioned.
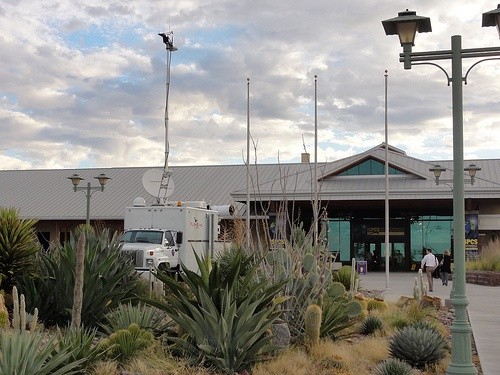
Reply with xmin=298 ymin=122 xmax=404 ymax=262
xmin=443 ymin=283 xmax=447 ymax=286
xmin=429 ymin=290 xmax=433 ymax=292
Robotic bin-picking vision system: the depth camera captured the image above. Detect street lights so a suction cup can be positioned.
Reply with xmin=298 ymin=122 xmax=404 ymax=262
xmin=67 ymin=172 xmax=108 ymax=226
xmin=381 ymin=2 xmax=500 ymax=374
xmin=430 ymin=162 xmax=480 ymax=186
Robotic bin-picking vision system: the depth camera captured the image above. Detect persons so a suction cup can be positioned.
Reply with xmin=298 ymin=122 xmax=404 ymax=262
xmin=438 ymin=249 xmax=451 ymax=286
xmin=421 ymin=248 xmax=440 ymax=292
xmin=364 ymin=250 xmax=380 ymax=270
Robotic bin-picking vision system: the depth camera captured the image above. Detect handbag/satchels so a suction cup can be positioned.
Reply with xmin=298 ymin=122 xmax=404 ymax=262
xmin=432 ymin=268 xmax=441 ymax=279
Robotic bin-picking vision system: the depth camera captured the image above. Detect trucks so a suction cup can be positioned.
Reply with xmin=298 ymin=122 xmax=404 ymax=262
xmin=116 ymin=198 xmax=235 ymax=278
